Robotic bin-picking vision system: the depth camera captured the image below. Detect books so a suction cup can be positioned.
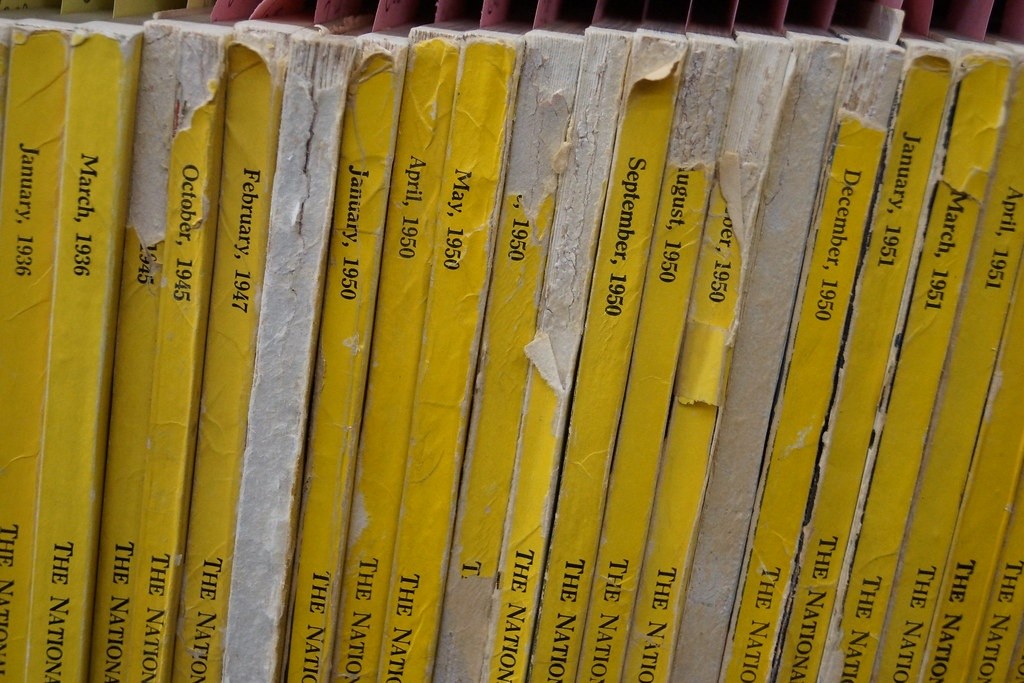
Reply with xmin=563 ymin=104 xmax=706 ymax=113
xmin=0 ymin=0 xmax=1024 ymax=682
